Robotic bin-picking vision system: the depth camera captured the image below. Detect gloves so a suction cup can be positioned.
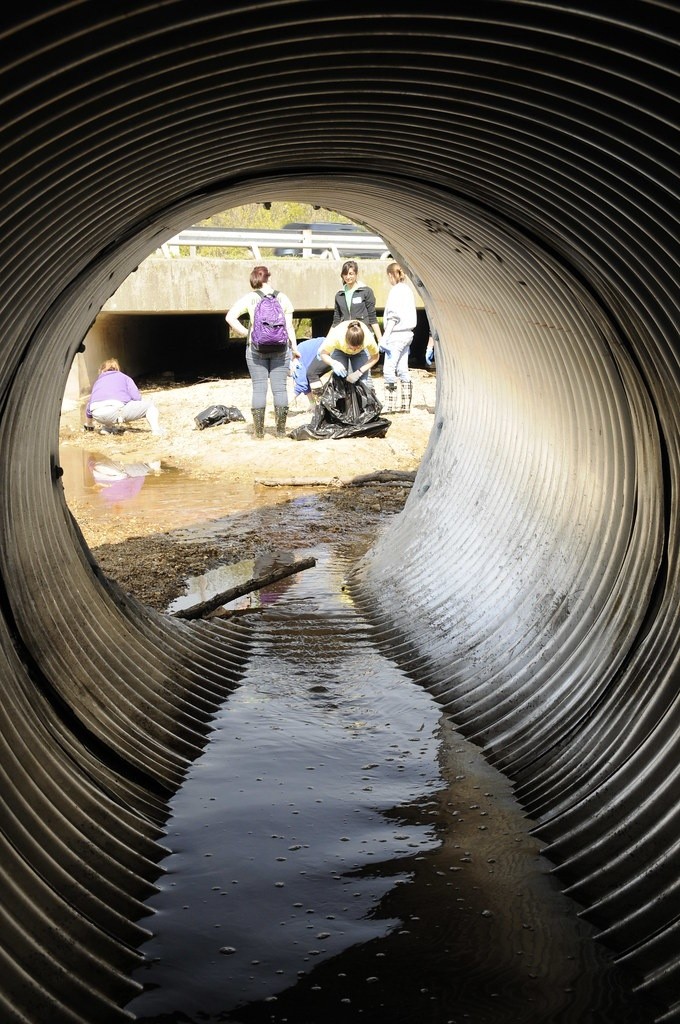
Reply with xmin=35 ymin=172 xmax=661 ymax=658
xmin=330 ymin=360 xmax=347 ymax=378
xmin=426 ymin=346 xmax=433 ymax=365
xmin=346 ymin=369 xmax=363 ymax=383
xmin=378 ymin=337 xmax=393 ymax=359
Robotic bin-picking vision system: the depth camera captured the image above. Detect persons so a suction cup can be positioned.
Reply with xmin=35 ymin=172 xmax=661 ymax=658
xmin=425 ymin=331 xmax=435 ymax=366
xmin=318 ymin=259 xmax=388 ymax=373
xmin=226 ymin=266 xmax=301 ymax=439
xmin=84 ymin=356 xmax=168 ymax=436
xmin=287 ymin=337 xmax=333 ymax=411
xmin=317 ymin=320 xmax=380 ymax=396
xmin=379 ymin=261 xmax=418 ymax=415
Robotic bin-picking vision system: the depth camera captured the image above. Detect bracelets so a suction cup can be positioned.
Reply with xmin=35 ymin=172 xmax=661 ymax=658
xmin=357 ymin=369 xmax=364 ymax=375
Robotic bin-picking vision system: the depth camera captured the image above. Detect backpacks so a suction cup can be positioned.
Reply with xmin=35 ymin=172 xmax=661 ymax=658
xmin=251 ymin=289 xmax=290 ymax=354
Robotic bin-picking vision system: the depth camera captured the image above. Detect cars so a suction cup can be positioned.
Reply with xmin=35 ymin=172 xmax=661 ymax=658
xmin=274 ymin=222 xmax=358 ymax=258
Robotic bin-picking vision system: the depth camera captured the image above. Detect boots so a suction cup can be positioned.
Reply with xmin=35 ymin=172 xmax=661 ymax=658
xmin=274 ymin=406 xmax=289 ymax=436
xmin=251 ymin=407 xmax=266 ymax=438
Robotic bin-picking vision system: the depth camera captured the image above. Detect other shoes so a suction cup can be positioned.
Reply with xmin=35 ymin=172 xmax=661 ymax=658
xmin=100 ymin=428 xmax=118 ymax=434
xmin=151 ymin=428 xmax=170 ymax=436
